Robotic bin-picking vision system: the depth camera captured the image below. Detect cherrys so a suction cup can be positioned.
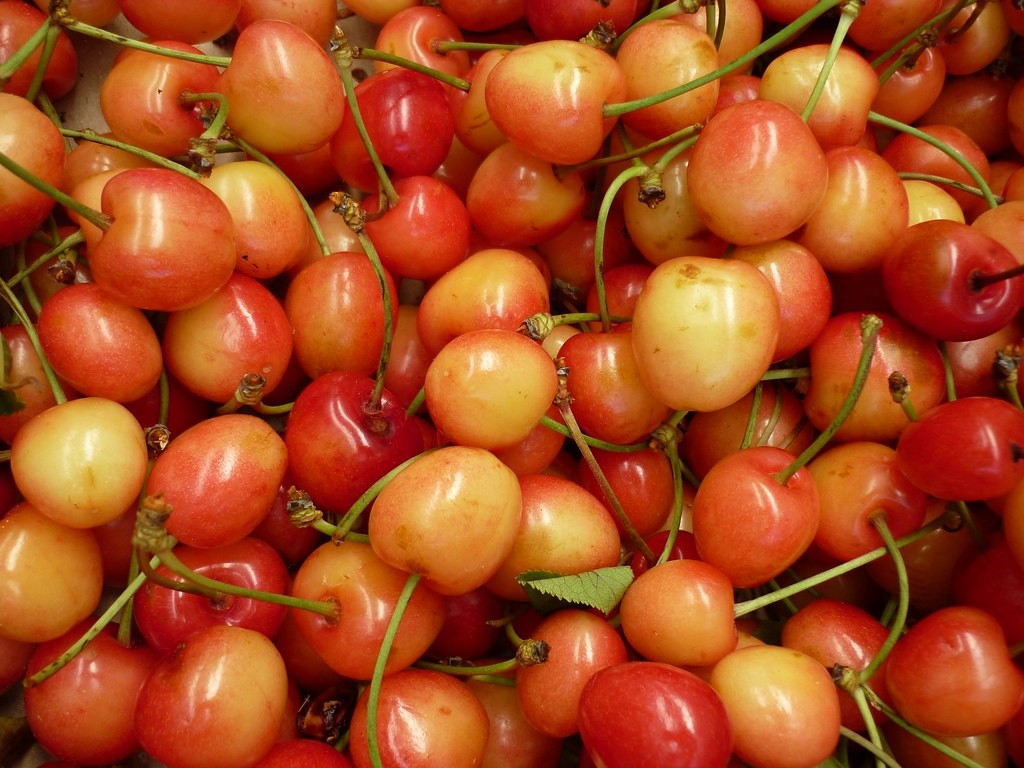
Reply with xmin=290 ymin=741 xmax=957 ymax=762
xmin=0 ymin=0 xmax=1024 ymax=768
